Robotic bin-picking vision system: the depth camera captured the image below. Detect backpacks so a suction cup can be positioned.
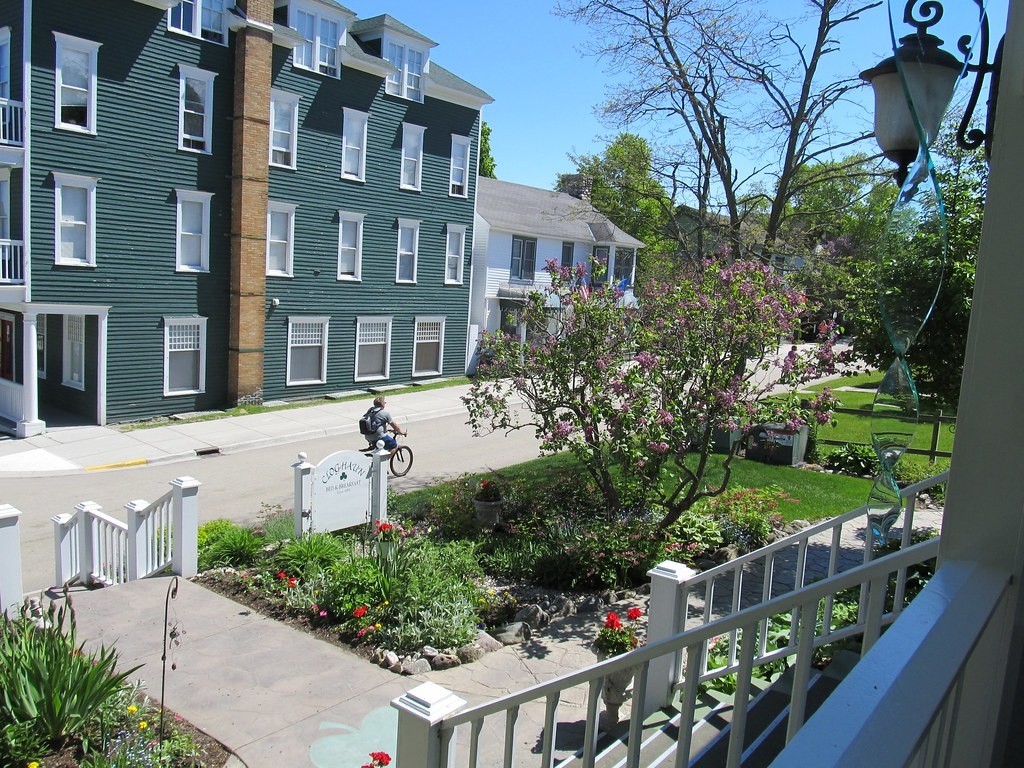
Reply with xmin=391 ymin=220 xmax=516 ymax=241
xmin=359 ymin=408 xmax=384 ymax=434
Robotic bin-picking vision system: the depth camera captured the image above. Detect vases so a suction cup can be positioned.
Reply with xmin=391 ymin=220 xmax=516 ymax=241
xmin=589 ymin=643 xmax=635 ymax=733
xmin=470 ymin=496 xmax=503 ymax=525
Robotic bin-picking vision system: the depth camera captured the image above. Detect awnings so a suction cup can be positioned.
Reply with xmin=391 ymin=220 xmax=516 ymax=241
xmin=501 ymin=283 xmax=642 ymax=310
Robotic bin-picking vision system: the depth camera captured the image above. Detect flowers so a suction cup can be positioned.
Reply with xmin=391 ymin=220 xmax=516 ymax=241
xmin=593 ymin=608 xmax=643 ymax=657
xmin=475 ymin=480 xmax=502 ymax=502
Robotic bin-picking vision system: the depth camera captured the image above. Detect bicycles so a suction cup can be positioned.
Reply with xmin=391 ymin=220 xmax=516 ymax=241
xmin=360 ymin=429 xmax=413 ymax=477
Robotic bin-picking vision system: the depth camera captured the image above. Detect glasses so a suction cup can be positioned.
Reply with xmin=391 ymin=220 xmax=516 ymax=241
xmin=383 ymin=401 xmax=386 ymax=404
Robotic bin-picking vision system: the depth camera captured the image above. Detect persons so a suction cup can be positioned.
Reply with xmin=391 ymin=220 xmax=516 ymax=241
xmin=364 ymin=397 xmax=407 ymax=461
xmin=819 ymin=320 xmax=829 ymax=337
xmin=786 ymin=346 xmax=804 ymax=373
xmin=828 ymin=316 xmax=835 ymax=327
